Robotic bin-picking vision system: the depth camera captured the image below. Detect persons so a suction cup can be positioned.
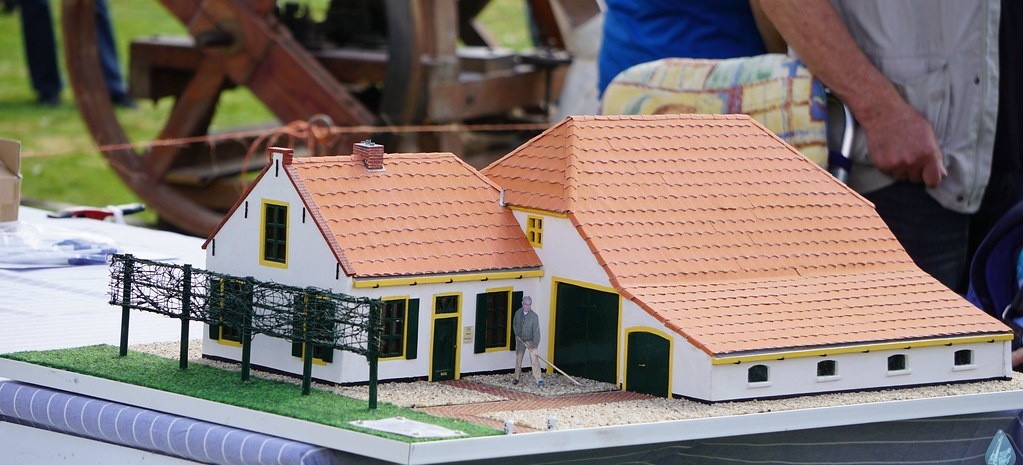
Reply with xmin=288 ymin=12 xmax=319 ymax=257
xmin=759 ymin=1 xmax=1023 ymax=321
xmin=599 ymin=1 xmax=764 ymax=92
xmin=17 ymin=0 xmax=139 ymax=108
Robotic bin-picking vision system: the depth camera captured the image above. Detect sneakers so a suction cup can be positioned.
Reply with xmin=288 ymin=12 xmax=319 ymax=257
xmin=512 ymin=378 xmax=518 ymax=385
xmin=537 ymin=380 xmax=544 ymax=387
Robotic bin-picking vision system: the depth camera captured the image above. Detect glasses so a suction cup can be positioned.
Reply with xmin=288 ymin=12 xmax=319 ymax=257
xmin=523 ymin=302 xmax=532 ymax=307
